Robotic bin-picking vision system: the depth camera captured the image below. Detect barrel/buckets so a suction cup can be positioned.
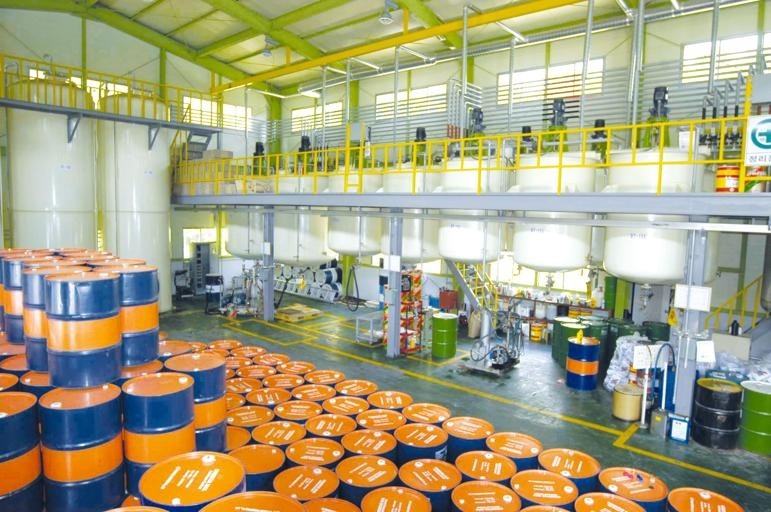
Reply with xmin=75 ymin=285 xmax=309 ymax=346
xmin=24 ymin=260 xmax=85 ymax=267
xmin=564 ymin=336 xmax=602 ymax=391
xmin=62 ymin=250 xmax=111 ymax=256
xmin=253 ymin=353 xmax=290 ymax=368
xmin=274 ymin=400 xmax=322 ymax=427
xmin=164 ymin=352 xmax=228 ymax=452
xmin=103 ymin=506 xmax=169 ymax=512
xmin=39 ymin=385 xmax=125 ymax=512
xmin=314 ymin=267 xmax=343 ymax=285
xmin=226 ymin=405 xmax=274 ymax=432
xmin=19 ymin=266 xmax=92 ymax=374
xmin=196 ymin=349 xmax=229 ymax=358
xmin=226 ymin=378 xmax=262 ymax=395
xmin=324 ymin=395 xmax=370 ymax=421
xmin=598 ymin=467 xmax=667 ymax=512
xmin=360 ymin=486 xmax=432 ymax=512
xmin=296 ymin=282 xmax=309 ymax=294
xmin=551 ymin=316 xmax=580 ymax=361
xmin=93 ymin=265 xmax=160 ymax=366
xmin=198 ymin=490 xmax=304 ymax=512
xmin=272 ymin=464 xmax=341 ymax=504
xmin=52 ymin=248 xmax=86 ymax=254
xmin=236 ymin=365 xmax=276 ymax=381
xmin=309 ymin=284 xmax=321 ymax=298
xmin=441 ymin=414 xmax=493 ymax=463
xmin=120 ymin=493 xmax=142 ymax=508
xmin=0 ymin=343 xmax=27 ymax=361
xmin=291 ymin=384 xmax=336 ymax=404
xmin=713 ymin=165 xmax=740 ymax=194
xmin=298 ymin=266 xmax=308 ymax=271
xmin=226 ymin=357 xmax=252 ymax=370
xmin=706 ymin=368 xmax=749 ymax=386
xmin=285 ymin=437 xmax=345 ymax=471
xmin=642 ymin=320 xmax=669 ymax=342
xmin=262 ymin=373 xmax=305 ymax=393
xmin=303 ymin=270 xmax=315 ymax=283
xmin=227 ymin=393 xmax=246 ymax=412
xmin=122 ymin=372 xmax=197 ymax=496
xmin=282 ymin=266 xmax=292 ymax=279
xmin=292 ymin=267 xmax=303 ymax=279
xmin=738 ymin=379 xmax=771 ymax=455
xmin=335 ymin=455 xmax=398 ymax=505
xmin=529 ymin=324 xmax=542 ymax=341
xmin=335 ymin=379 xmax=378 ymax=401
xmin=226 ymin=368 xmax=236 ymax=380
xmin=2 ymin=256 xmax=64 ymax=345
xmin=520 ymin=505 xmax=570 ymax=512
xmin=368 ymin=389 xmax=412 ymax=414
xmin=204 ymin=273 xmax=224 ymax=303
xmin=452 ymin=481 xmax=522 ymax=512
xmin=321 ymin=282 xmax=343 ymax=301
xmin=287 ymin=279 xmax=298 ymax=293
xmin=122 ymin=361 xmax=163 ymax=386
xmin=188 ymin=342 xmax=207 ymax=352
xmin=665 ymin=486 xmax=744 ymax=512
xmin=87 ymin=257 xmax=147 ymax=268
xmin=538 ymin=447 xmax=600 ymax=492
xmin=558 ymin=324 xmax=587 ymax=369
xmin=399 ymin=457 xmax=462 ymax=512
xmin=511 ymin=469 xmax=578 ymax=512
xmin=231 ymin=345 xmax=266 ymax=359
xmin=486 ymin=431 xmax=541 ymax=471
xmin=579 ymin=314 xmax=605 ymax=322
xmin=0 ymin=251 xmax=52 ymax=333
xmin=393 ymin=423 xmax=449 ymax=465
xmin=247 ymin=387 xmax=291 ymax=410
xmin=276 ymin=360 xmax=316 ymax=378
xmin=617 ymin=324 xmax=643 ymax=338
xmin=158 ymin=340 xmax=192 ymax=373
xmin=342 ymin=429 xmax=397 ymax=463
xmin=311 ymin=259 xmax=338 ymax=272
xmin=44 ymin=272 xmax=122 ymax=390
xmin=304 ymin=369 xmax=345 ymax=388
xmin=0 ymin=373 xmax=19 ymax=392
xmin=138 ymin=450 xmax=247 ymax=512
xmin=572 ymin=491 xmax=647 ymax=512
xmin=456 ymin=450 xmax=516 ymax=488
xmin=403 ymin=401 xmax=451 ymax=428
xmin=1 ymin=332 xmax=7 ymax=344
xmin=305 ymin=497 xmax=360 ymax=512
xmin=224 ymin=425 xmax=251 ymax=453
xmin=251 ymin=420 xmax=307 ymax=450
xmin=357 ymin=408 xmax=406 ymax=433
xmin=304 ymin=414 xmax=357 ymax=442
xmin=581 ymin=320 xmax=609 ymax=363
xmin=439 ymin=289 xmax=459 ymax=310
xmin=207 ymin=340 xmax=242 ymax=351
xmin=431 ymin=313 xmax=459 ymax=360
xmin=608 ymin=317 xmax=633 ymax=352
xmin=273 ymin=265 xmax=281 ymax=278
xmin=18 ymin=369 xmax=53 ymax=398
xmin=0 ymin=354 xmax=29 ymax=375
xmin=65 ymin=255 xmax=116 ymax=261
xmin=0 ymin=391 xmax=43 ymax=512
xmin=160 ymin=332 xmax=168 ymax=340
xmin=691 ymin=377 xmax=743 ymax=452
xmin=228 ymin=443 xmax=285 ymax=492
xmin=275 ymin=277 xmax=285 ymax=290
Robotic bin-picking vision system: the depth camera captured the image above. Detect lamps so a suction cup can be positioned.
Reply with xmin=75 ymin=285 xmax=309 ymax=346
xmin=261 ymin=36 xmax=279 ymax=58
xmin=377 ymin=1 xmax=398 ymax=25
